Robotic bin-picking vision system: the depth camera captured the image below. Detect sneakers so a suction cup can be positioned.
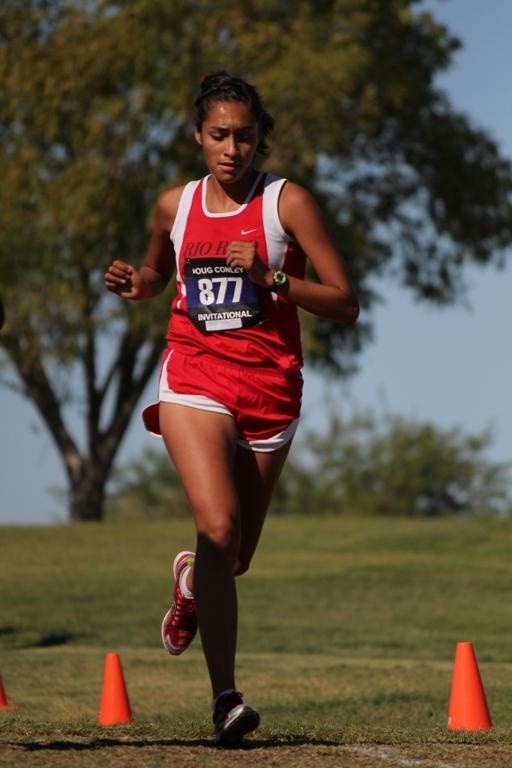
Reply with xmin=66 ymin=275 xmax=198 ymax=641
xmin=162 ymin=550 xmax=199 ymax=654
xmin=212 ymin=689 xmax=261 ymax=746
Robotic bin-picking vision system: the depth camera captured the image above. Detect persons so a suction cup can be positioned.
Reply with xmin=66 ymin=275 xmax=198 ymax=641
xmin=100 ymin=69 xmax=362 ymax=748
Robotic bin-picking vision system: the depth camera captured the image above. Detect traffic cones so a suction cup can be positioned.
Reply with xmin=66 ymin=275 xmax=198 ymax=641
xmin=0 ymin=673 xmax=22 ymax=711
xmin=444 ymin=639 xmax=495 ymax=735
xmin=90 ymin=648 xmax=136 ymax=726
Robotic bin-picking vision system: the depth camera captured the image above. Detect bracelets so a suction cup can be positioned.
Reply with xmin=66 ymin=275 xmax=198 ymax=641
xmin=270 ymin=267 xmax=289 ymax=296
